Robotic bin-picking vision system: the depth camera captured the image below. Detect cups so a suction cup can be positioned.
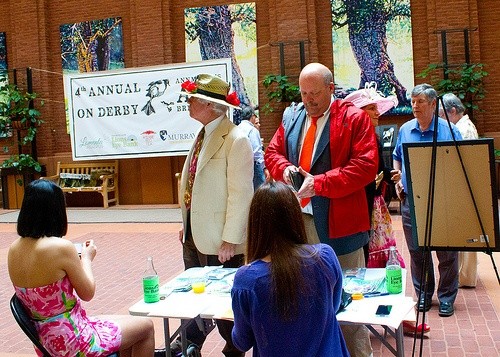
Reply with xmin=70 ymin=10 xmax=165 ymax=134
xmin=190 ymin=276 xmax=206 ymax=294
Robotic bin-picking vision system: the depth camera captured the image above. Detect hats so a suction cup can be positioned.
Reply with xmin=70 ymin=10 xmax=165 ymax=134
xmin=344 ymin=89 xmax=395 ymax=116
xmin=180 ymin=74 xmax=242 ymax=110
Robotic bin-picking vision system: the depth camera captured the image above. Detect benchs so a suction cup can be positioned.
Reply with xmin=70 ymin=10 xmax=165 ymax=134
xmin=40 ymin=160 xmax=119 ymax=208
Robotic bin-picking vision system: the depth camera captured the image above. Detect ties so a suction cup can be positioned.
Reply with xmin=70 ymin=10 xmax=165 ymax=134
xmin=298 ymin=117 xmax=322 ymax=208
xmin=184 ymin=127 xmax=205 ymax=209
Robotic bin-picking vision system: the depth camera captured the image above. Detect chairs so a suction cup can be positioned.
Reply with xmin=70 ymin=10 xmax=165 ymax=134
xmin=10 ymin=291 xmax=120 ymax=357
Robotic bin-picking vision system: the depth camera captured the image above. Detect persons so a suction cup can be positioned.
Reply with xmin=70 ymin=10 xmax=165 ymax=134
xmin=8 ymin=179 xmax=155 ymax=357
xmin=438 ymin=93 xmax=479 ymax=287
xmin=231 ymin=182 xmax=350 ymax=357
xmin=263 ymin=63 xmax=378 ymax=357
xmin=154 ymin=75 xmax=254 ymax=357
xmin=238 ymin=106 xmax=270 ymax=189
xmin=344 ymin=89 xmax=430 ymax=334
xmin=392 ymin=84 xmax=462 ymax=315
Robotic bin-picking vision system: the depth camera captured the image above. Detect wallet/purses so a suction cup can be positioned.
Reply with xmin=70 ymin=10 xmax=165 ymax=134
xmin=289 ymin=170 xmax=304 ymax=192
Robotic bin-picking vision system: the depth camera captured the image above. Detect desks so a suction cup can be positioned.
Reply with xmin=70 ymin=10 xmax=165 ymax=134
xmin=129 ymin=267 xmax=405 ymax=357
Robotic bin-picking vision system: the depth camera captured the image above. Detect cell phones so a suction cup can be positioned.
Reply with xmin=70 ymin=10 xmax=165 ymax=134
xmin=375 ymin=305 xmax=392 ymax=317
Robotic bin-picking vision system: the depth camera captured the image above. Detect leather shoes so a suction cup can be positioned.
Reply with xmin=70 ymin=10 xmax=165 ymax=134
xmin=439 ymin=301 xmax=454 ymax=315
xmin=416 ymin=296 xmax=433 ymax=310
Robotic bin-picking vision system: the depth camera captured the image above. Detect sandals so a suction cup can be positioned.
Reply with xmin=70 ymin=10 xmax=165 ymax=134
xmin=402 ymin=322 xmax=430 ymax=335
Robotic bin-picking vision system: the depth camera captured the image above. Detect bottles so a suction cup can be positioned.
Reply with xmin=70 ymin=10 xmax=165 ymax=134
xmin=385 ymin=246 xmax=402 ymax=295
xmin=142 ymin=256 xmax=160 ymax=303
